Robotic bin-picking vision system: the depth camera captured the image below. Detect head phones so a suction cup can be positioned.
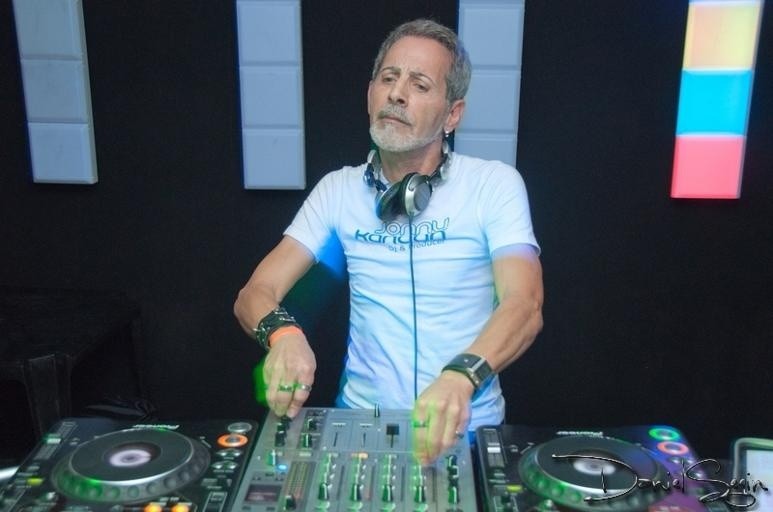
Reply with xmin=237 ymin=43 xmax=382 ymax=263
xmin=363 ymin=138 xmax=452 ymax=221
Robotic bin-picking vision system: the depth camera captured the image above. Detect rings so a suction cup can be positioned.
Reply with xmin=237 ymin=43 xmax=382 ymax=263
xmin=295 ymin=381 xmax=311 ymax=394
xmin=276 ymin=382 xmax=293 ymax=391
xmin=451 ymin=430 xmax=466 ymax=440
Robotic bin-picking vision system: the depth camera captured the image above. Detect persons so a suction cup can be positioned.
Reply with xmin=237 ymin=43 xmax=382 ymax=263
xmin=231 ymin=19 xmax=544 ymax=466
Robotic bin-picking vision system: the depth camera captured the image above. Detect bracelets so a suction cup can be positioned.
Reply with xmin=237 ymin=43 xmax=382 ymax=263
xmin=440 ymin=351 xmax=494 ymax=402
xmin=248 ymin=307 xmax=301 ymax=350
